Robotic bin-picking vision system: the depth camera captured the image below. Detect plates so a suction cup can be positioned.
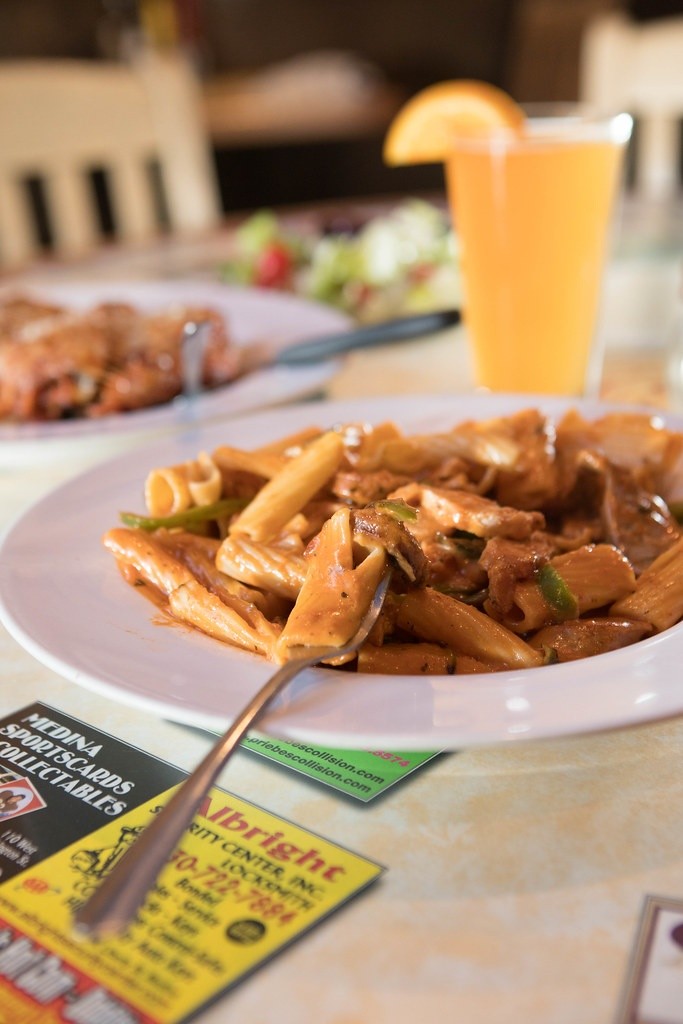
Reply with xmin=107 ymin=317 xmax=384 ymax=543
xmin=0 ymin=394 xmax=683 ymax=751
xmin=1 ymin=281 xmax=356 ymax=439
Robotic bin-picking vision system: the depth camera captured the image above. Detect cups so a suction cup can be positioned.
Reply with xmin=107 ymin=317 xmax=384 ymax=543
xmin=448 ymin=113 xmax=632 ymax=402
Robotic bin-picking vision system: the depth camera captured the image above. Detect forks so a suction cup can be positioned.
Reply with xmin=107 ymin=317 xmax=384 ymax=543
xmin=77 ymin=572 xmax=390 ymax=934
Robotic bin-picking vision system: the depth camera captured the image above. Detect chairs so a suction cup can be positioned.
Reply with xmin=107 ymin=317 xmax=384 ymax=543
xmin=0 ymin=40 xmax=221 ymax=274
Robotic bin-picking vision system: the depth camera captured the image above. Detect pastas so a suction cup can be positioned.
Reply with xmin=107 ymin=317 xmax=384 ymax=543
xmin=102 ymin=411 xmax=683 ymax=673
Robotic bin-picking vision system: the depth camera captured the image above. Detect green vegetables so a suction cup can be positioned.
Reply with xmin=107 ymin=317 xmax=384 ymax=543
xmin=120 ymin=494 xmax=579 ymax=623
